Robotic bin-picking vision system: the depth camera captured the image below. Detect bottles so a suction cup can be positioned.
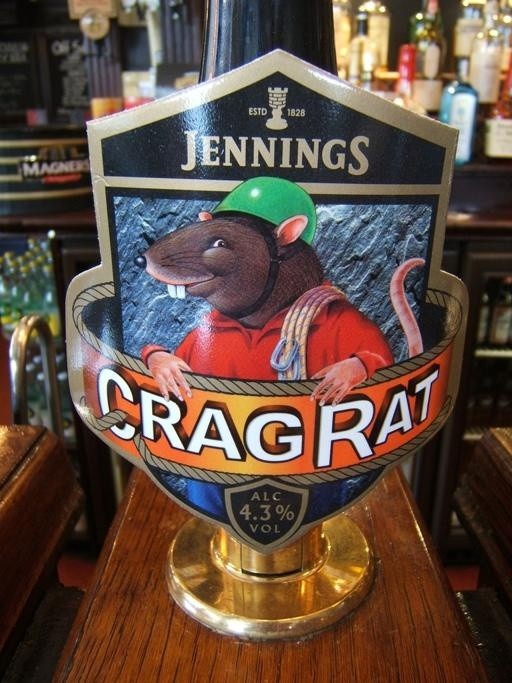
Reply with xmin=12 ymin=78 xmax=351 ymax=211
xmin=1 ymin=237 xmax=77 ymax=444
xmin=441 ymin=81 xmax=475 ymax=164
xmin=346 ymin=13 xmax=373 ymax=92
xmin=469 ymin=2 xmax=502 ymax=104
xmin=391 ymin=43 xmax=427 ymax=117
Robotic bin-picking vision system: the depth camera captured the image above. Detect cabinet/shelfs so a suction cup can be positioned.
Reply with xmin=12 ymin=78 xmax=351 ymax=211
xmin=0 ymin=160 xmax=512 ymax=682
